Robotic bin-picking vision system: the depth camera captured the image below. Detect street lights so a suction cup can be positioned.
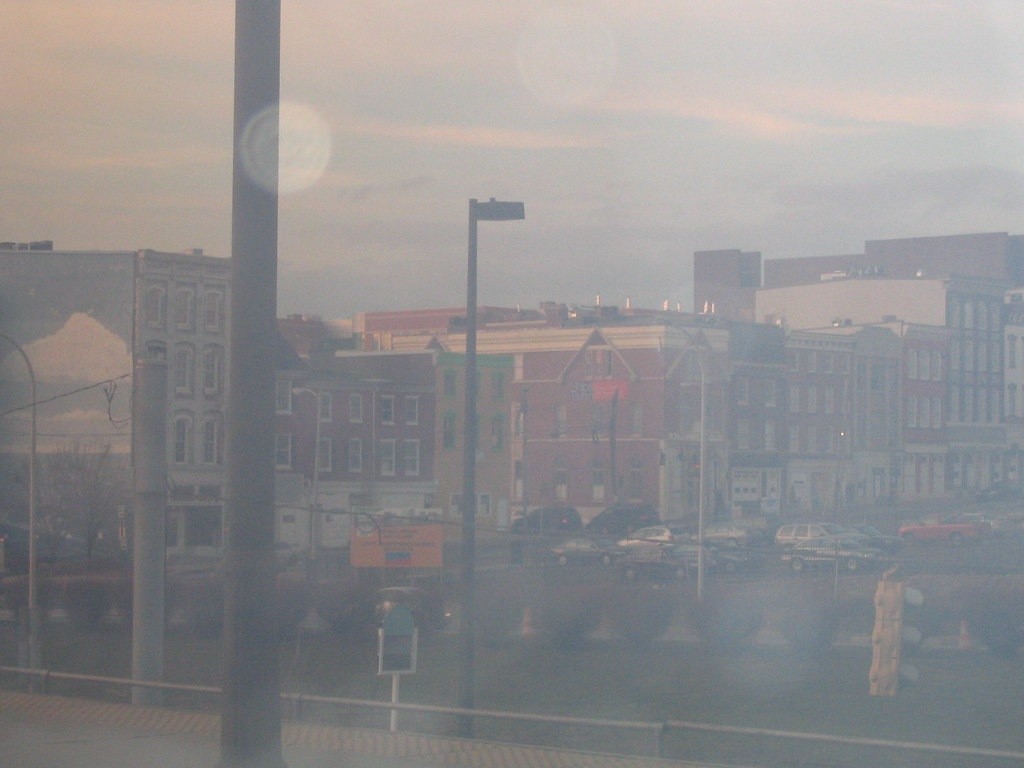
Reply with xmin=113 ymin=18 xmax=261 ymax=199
xmin=463 ymin=197 xmax=526 ymax=737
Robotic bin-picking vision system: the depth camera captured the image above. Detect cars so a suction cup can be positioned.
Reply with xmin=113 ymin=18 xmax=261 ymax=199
xmin=550 ymin=511 xmax=1010 ymax=582
